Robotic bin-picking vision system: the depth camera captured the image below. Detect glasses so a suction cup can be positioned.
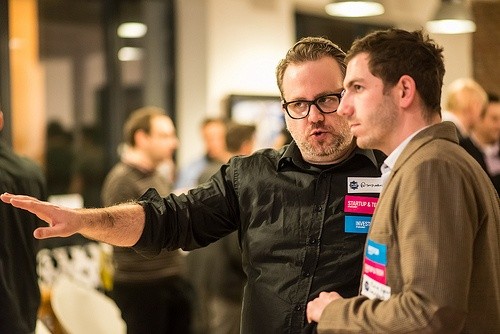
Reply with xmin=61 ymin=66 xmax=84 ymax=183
xmin=282 ymin=89 xmax=346 ymax=119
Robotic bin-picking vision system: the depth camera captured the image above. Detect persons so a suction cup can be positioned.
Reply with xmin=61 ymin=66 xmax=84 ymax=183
xmin=306 ymin=28 xmax=500 ymax=334
xmin=0 ymin=111 xmax=49 ymax=334
xmin=101 ymin=76 xmax=500 ymax=334
xmin=1 ymin=35 xmax=389 ymax=333
